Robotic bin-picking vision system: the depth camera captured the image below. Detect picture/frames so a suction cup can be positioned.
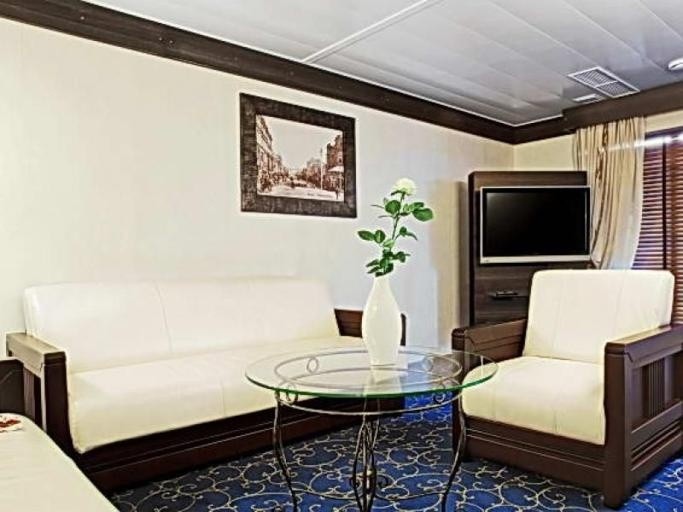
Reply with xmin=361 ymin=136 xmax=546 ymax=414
xmin=239 ymin=91 xmax=357 ymax=219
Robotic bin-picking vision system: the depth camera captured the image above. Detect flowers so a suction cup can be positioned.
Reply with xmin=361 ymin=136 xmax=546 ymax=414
xmin=359 ymin=177 xmax=434 ymax=277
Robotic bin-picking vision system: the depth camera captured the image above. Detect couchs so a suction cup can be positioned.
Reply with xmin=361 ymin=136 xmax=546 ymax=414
xmin=6 ymin=279 xmax=406 ymax=490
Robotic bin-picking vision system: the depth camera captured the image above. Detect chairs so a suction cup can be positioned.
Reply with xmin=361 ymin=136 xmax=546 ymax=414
xmin=452 ymin=269 xmax=683 ymax=512
xmin=0 ymin=413 xmax=118 ymax=512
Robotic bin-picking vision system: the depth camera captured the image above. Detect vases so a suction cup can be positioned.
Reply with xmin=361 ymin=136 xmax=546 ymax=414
xmin=360 ymin=274 xmax=403 ymax=366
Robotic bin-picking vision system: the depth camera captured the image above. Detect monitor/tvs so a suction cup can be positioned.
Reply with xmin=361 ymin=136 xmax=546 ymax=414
xmin=478 ymin=185 xmax=594 ymax=264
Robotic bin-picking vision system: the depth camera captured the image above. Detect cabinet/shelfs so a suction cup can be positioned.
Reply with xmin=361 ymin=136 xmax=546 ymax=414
xmin=468 ymin=171 xmax=593 ymax=324
